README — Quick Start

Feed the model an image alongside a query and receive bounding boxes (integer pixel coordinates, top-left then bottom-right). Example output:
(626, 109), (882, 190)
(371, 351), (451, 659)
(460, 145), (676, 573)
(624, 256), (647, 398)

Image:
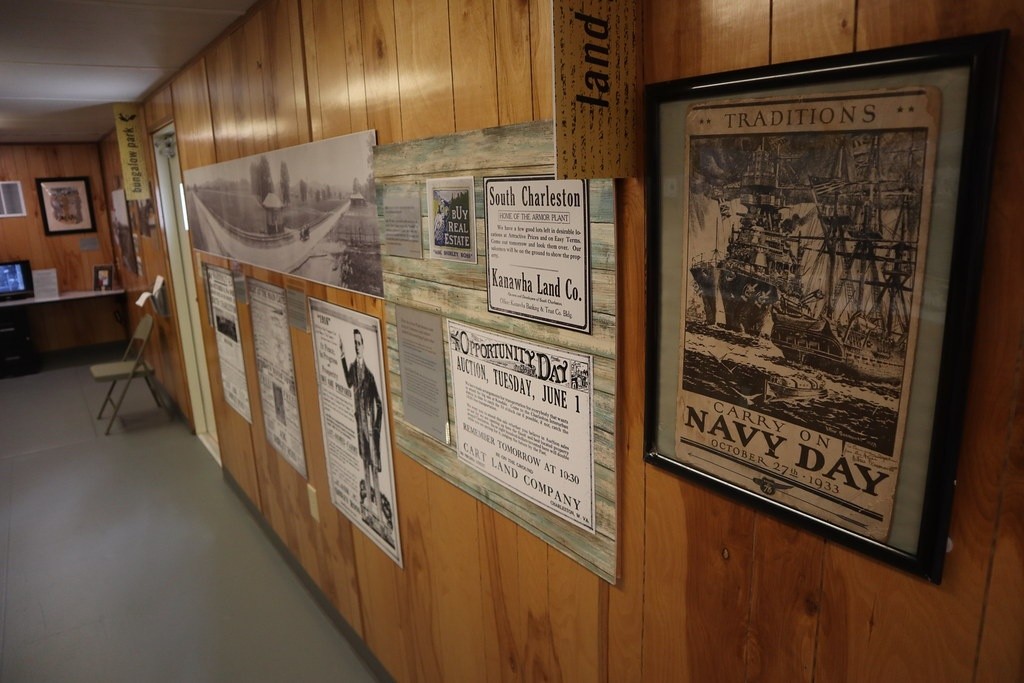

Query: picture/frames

(641, 27), (1012, 586)
(93, 265), (114, 292)
(34, 176), (98, 236)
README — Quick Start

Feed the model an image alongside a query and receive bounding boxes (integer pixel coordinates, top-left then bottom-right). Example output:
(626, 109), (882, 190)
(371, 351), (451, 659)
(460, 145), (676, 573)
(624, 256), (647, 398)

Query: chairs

(88, 312), (175, 437)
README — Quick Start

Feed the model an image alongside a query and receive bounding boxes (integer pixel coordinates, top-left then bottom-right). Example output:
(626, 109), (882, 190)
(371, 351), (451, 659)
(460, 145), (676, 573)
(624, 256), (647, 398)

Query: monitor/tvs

(0, 259), (34, 302)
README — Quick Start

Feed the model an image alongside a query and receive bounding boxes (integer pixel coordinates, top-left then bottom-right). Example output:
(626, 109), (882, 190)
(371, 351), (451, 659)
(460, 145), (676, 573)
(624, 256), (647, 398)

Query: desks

(0, 283), (125, 308)
